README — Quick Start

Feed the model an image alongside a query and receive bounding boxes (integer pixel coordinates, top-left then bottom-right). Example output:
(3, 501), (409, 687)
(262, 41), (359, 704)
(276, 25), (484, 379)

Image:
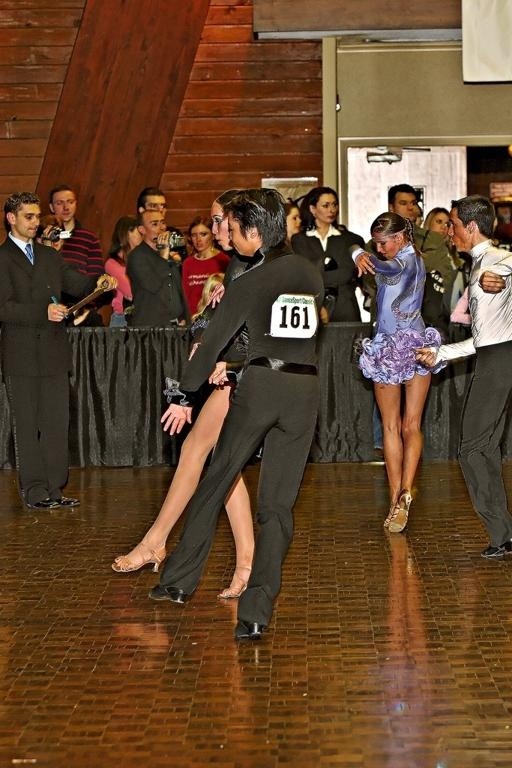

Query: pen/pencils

(51, 296), (69, 320)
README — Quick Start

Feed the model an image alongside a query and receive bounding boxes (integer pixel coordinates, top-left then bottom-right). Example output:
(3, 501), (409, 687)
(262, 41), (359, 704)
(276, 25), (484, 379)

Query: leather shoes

(480, 537), (512, 558)
(148, 583), (190, 604)
(55, 496), (81, 507)
(235, 619), (264, 640)
(27, 497), (61, 509)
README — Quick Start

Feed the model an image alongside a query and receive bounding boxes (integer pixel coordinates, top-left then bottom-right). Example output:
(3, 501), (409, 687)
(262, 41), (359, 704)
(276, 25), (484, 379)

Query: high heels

(219, 567), (252, 599)
(111, 541), (167, 573)
(388, 489), (412, 533)
(383, 503), (409, 529)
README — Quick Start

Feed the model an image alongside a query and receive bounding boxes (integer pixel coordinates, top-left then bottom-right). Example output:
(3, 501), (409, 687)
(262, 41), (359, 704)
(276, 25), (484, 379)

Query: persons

(282, 184), (474, 325)
(413, 194), (512, 558)
(111, 189), (255, 599)
(1, 192), (81, 510)
(348, 213), (447, 534)
(146, 188), (325, 642)
(38, 187), (230, 327)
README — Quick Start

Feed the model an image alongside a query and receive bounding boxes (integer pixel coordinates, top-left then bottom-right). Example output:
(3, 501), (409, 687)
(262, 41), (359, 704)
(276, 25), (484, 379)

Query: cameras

(49, 226), (71, 242)
(168, 231), (186, 249)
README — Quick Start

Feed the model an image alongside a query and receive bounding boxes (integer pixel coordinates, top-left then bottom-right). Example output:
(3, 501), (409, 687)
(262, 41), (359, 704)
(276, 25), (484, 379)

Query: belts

(249, 357), (318, 375)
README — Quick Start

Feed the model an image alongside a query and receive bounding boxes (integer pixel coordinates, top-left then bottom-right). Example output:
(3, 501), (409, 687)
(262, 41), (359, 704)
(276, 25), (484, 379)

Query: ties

(25, 244), (33, 265)
